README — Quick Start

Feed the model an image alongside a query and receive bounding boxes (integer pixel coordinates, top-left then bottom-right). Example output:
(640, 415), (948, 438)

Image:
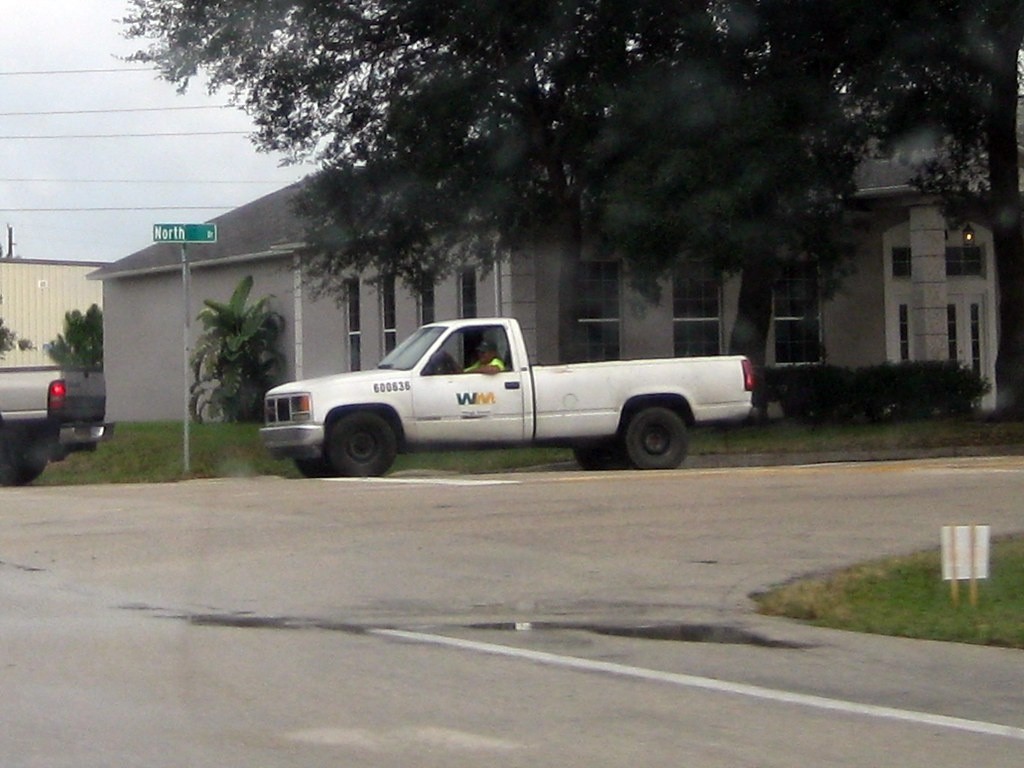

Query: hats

(476, 339), (496, 352)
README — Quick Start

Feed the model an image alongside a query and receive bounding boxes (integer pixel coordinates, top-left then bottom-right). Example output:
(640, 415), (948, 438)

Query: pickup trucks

(0, 366), (105, 485)
(259, 317), (755, 477)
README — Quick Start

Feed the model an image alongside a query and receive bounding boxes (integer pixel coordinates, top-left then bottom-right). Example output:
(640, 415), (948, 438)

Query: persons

(449, 340), (505, 374)
